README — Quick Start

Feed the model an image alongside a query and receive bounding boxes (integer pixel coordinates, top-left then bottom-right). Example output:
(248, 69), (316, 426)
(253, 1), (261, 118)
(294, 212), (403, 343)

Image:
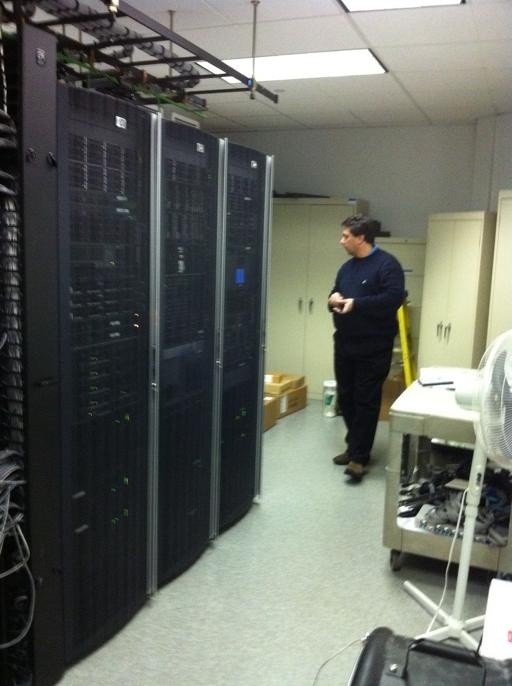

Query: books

(417, 373), (455, 386)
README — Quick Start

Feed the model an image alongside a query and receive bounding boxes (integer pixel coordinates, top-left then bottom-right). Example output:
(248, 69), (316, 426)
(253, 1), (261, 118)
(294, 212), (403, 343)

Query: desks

(380, 367), (511, 580)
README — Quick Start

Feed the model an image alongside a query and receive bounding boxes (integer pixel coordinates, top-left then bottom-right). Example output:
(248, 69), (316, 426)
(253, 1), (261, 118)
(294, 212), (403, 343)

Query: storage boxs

(263, 372), (307, 435)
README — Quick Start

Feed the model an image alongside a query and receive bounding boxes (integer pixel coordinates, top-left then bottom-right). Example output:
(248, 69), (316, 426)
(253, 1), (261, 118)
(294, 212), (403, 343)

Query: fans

(401, 328), (512, 650)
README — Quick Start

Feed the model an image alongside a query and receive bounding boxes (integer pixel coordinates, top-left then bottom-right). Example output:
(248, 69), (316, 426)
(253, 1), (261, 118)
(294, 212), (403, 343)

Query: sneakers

(345, 460), (369, 478)
(333, 452), (370, 465)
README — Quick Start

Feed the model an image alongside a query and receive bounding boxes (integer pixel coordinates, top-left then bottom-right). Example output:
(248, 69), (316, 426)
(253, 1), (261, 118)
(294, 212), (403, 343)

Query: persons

(326, 213), (408, 482)
